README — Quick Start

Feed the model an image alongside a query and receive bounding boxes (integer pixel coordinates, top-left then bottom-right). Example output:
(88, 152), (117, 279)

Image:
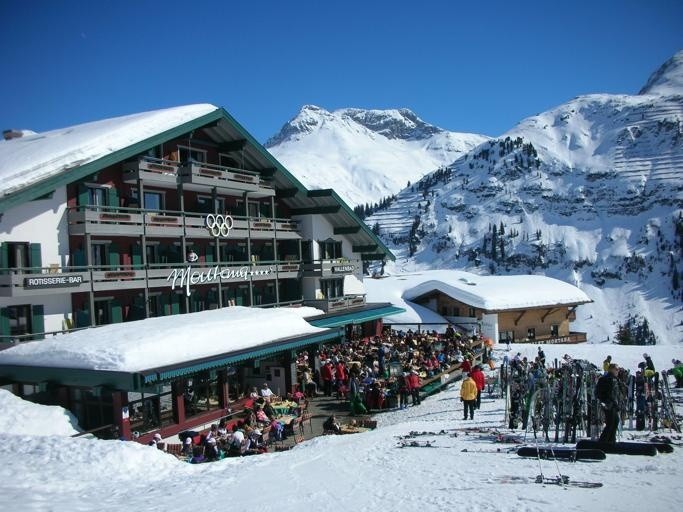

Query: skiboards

(394, 427), (532, 454)
(487, 472), (603, 489)
(499, 358), (682, 443)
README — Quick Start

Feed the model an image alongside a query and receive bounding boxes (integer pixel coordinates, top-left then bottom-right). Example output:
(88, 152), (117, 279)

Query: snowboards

(576, 440), (659, 456)
(517, 447), (606, 460)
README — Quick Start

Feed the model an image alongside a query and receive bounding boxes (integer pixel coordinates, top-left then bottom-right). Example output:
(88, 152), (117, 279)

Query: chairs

(322, 420), (377, 434)
(156, 391), (313, 460)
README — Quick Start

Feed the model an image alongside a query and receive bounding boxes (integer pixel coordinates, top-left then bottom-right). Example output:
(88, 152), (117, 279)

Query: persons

(510, 347), (683, 442)
(133, 383), (304, 463)
(297, 323), (496, 416)
(187, 248), (198, 267)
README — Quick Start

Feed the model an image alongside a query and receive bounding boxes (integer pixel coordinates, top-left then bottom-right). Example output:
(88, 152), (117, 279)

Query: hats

(186, 437), (192, 445)
(208, 438), (216, 443)
(154, 434), (162, 441)
(254, 430), (262, 436)
